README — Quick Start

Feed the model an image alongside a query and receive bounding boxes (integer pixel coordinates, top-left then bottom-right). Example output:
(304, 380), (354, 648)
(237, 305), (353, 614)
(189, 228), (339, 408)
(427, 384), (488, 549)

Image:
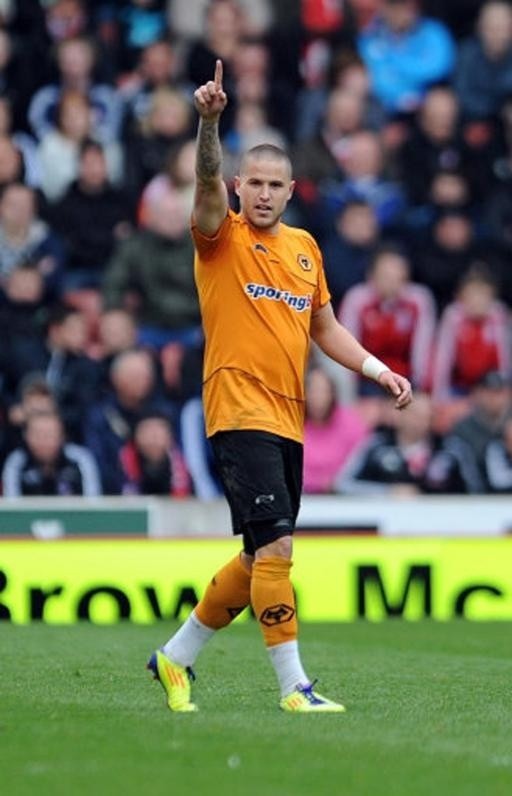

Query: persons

(148, 59), (414, 713)
(1, 1), (511, 497)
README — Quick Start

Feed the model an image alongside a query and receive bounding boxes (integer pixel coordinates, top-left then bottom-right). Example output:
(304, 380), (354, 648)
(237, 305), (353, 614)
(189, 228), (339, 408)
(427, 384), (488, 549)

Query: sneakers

(146, 650), (197, 713)
(279, 687), (345, 714)
